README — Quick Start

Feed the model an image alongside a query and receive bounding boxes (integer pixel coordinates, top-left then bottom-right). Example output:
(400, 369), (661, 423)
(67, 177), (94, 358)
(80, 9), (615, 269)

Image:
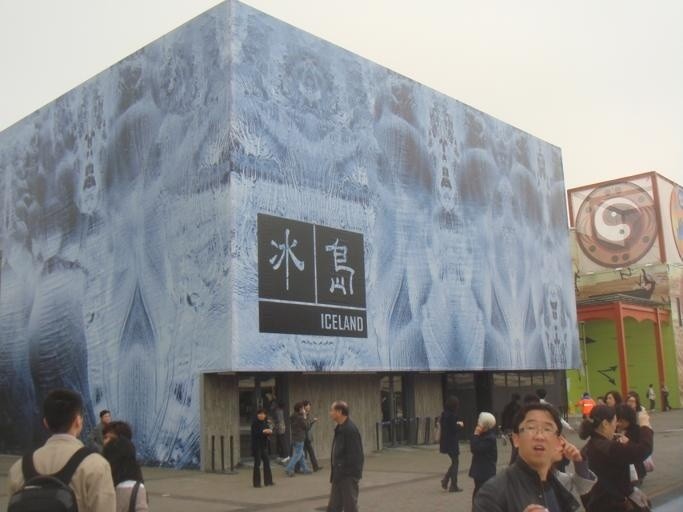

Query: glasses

(519, 425), (560, 437)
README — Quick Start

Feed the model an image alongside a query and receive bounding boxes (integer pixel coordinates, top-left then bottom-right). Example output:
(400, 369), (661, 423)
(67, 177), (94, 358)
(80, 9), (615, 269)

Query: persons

(249, 408), (275, 487)
(325, 399), (364, 512)
(283, 402), (316, 477)
(9, 387), (116, 512)
(268, 387), (291, 464)
(87, 410), (112, 452)
(439, 381), (674, 511)
(99, 420), (132, 458)
(293, 400), (324, 473)
(101, 435), (146, 512)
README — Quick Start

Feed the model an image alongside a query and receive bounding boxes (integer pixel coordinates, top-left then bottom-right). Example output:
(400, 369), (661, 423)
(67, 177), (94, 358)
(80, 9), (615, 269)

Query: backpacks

(6, 446), (96, 511)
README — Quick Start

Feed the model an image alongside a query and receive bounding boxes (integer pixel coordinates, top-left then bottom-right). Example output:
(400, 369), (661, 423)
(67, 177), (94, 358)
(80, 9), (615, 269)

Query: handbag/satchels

(623, 490), (650, 512)
